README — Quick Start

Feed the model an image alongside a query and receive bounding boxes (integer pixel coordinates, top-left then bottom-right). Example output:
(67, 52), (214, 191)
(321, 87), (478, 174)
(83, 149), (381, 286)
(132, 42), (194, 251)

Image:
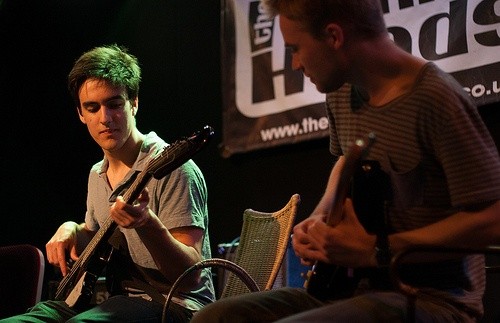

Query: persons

(0, 43), (217, 323)
(187, 0), (500, 323)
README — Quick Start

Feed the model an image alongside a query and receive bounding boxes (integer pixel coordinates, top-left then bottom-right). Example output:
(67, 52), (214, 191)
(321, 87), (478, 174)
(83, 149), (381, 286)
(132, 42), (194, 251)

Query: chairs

(162, 193), (301, 323)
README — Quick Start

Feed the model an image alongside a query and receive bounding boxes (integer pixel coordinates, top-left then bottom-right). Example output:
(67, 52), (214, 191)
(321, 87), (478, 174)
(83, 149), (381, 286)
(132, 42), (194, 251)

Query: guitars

(54, 126), (215, 308)
(303, 133), (385, 300)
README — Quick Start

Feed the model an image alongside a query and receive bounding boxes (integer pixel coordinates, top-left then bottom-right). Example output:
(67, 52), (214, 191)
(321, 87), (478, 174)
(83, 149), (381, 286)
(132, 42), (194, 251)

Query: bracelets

(376, 234), (393, 266)
